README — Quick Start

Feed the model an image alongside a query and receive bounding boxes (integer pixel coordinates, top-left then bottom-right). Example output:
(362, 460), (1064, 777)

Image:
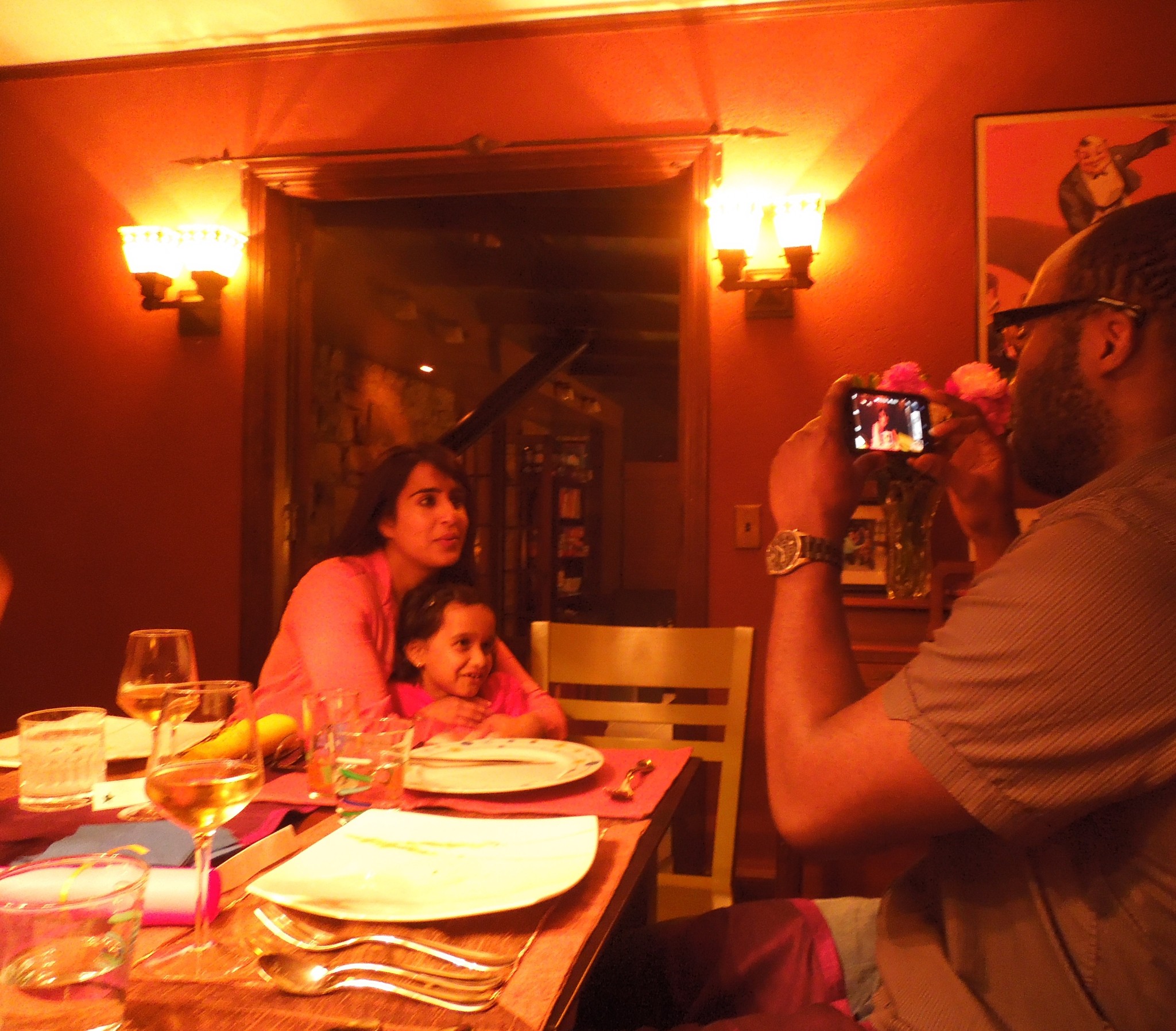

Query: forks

(253, 898), (518, 969)
(607, 758), (654, 799)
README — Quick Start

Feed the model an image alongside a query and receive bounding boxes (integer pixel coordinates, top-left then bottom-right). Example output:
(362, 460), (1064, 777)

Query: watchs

(765, 529), (842, 576)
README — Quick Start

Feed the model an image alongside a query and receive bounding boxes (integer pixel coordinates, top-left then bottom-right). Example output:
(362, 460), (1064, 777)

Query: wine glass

(135, 679), (266, 980)
(114, 630), (202, 793)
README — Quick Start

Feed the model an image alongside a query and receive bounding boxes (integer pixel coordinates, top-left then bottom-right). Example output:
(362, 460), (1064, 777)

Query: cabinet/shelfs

(522, 434), (603, 626)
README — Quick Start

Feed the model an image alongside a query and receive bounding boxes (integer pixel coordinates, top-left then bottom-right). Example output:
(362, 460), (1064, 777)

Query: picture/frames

(974, 100), (1176, 364)
(838, 498), (890, 596)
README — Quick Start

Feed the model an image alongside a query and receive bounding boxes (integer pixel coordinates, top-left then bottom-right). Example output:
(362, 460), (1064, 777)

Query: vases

(886, 474), (943, 593)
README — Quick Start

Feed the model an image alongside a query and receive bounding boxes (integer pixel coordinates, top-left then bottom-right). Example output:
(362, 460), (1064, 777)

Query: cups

(1, 854), (146, 1031)
(14, 706), (106, 811)
(335, 716), (417, 806)
(301, 690), (362, 803)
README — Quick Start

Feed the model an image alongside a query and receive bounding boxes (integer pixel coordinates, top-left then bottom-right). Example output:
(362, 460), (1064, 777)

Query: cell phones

(844, 388), (933, 461)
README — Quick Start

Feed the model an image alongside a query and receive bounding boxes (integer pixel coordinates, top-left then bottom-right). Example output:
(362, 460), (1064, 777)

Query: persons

(577, 190), (1176, 1031)
(221, 442), (568, 745)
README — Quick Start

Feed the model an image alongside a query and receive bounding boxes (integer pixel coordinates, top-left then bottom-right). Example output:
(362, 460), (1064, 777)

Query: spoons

(255, 951), (505, 1015)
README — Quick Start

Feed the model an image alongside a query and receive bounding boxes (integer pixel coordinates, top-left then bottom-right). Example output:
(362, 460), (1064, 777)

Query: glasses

(987, 296), (1141, 381)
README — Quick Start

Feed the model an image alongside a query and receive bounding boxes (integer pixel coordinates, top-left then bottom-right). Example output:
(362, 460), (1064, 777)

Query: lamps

(703, 180), (824, 323)
(117, 223), (252, 335)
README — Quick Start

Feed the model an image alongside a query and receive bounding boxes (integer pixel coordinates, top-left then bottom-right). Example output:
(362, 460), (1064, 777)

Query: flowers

(862, 352), (1011, 585)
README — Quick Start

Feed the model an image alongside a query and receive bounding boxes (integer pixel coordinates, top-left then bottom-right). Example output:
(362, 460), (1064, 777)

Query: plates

(0, 711), (227, 764)
(245, 807), (601, 926)
(400, 737), (605, 793)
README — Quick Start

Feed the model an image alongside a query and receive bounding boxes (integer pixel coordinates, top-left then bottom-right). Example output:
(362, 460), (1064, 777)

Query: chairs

(528, 621), (756, 925)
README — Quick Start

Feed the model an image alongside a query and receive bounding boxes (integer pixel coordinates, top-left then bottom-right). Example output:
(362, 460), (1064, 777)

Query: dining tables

(0, 711), (701, 1031)
